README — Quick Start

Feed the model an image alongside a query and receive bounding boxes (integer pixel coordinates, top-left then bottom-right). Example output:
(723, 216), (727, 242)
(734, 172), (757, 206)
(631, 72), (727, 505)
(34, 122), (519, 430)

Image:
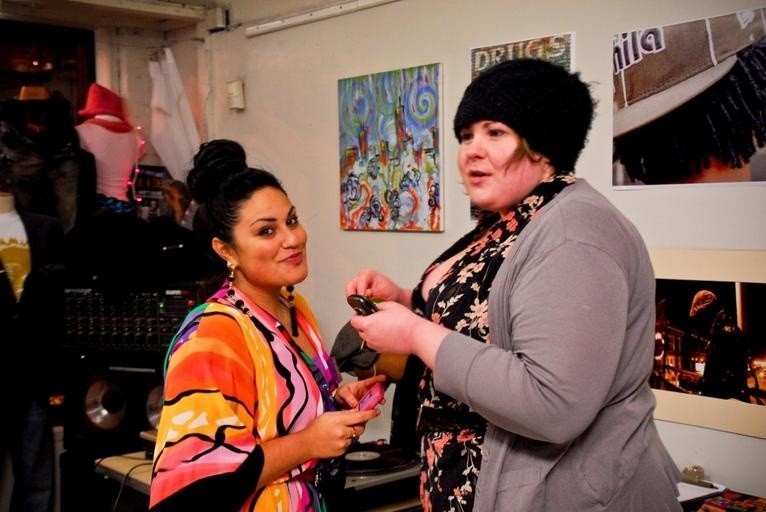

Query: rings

(351, 426), (358, 440)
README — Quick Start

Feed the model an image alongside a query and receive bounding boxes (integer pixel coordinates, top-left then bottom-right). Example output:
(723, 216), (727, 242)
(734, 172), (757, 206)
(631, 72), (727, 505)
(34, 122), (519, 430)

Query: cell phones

(347, 293), (377, 314)
(354, 381), (384, 414)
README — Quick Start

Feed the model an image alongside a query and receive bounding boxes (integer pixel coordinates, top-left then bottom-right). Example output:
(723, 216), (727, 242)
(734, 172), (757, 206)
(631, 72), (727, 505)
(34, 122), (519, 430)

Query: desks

(92, 429), (424, 512)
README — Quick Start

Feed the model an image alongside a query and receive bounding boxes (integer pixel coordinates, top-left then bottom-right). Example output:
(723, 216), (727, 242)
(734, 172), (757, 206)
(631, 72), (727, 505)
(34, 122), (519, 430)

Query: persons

(680, 291), (751, 401)
(148, 137), (389, 512)
(160, 140), (247, 300)
(345, 58), (684, 512)
(615, 6), (765, 185)
(0, 82), (144, 510)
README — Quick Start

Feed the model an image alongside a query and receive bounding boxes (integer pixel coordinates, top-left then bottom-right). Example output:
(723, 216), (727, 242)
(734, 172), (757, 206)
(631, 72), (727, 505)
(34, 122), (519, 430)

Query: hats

(689, 289), (717, 317)
(77, 83), (125, 121)
(453, 58), (593, 171)
